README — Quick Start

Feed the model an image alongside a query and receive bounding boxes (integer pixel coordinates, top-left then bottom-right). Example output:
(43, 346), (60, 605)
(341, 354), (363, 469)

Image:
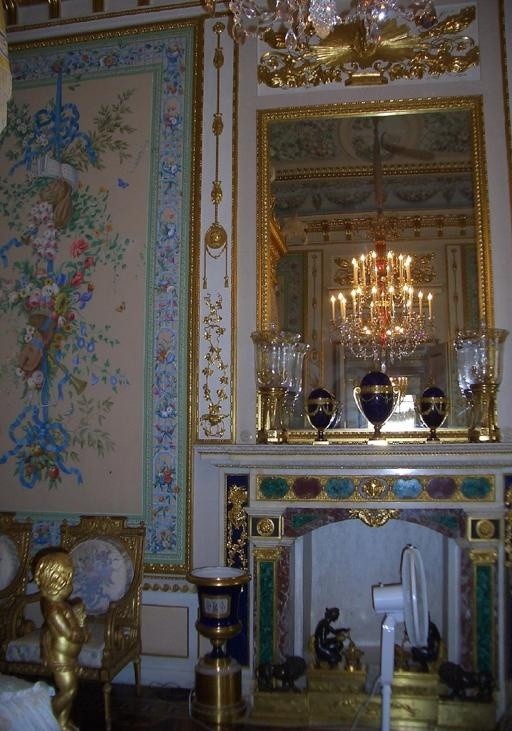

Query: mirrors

(255, 95), (500, 443)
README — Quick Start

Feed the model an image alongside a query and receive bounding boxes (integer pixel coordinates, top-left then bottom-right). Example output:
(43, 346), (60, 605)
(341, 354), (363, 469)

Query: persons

(315, 607), (346, 668)
(31, 551), (89, 731)
(405, 612), (441, 673)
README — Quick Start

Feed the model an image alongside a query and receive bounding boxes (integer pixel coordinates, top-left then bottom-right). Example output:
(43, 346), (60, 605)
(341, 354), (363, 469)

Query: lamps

(328, 117), (439, 374)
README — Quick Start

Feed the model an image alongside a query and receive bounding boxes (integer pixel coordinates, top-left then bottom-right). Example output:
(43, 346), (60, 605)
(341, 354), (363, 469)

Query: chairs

(0, 515), (146, 731)
(0, 512), (34, 646)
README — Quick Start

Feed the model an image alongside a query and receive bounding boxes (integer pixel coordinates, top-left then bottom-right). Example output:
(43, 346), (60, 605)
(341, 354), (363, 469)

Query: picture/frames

(0, 15), (205, 577)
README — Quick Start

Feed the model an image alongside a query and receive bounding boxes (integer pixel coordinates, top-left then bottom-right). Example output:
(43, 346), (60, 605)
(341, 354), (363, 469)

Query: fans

(372, 543), (430, 731)
